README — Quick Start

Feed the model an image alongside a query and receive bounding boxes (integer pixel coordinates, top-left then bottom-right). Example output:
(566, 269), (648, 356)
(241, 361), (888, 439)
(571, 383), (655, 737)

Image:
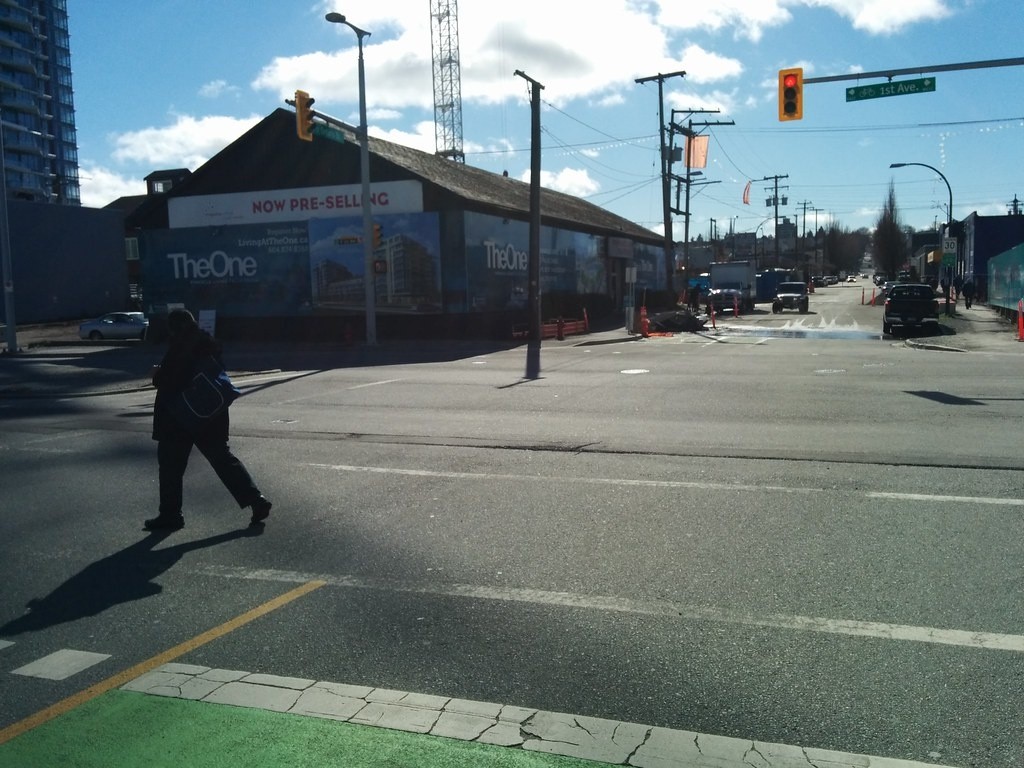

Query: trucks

(707, 261), (756, 314)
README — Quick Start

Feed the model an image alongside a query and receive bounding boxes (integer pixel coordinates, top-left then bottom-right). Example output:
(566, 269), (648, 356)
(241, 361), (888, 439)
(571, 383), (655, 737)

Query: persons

(143, 309), (272, 530)
(941, 275), (951, 294)
(929, 276), (938, 291)
(689, 283), (701, 311)
(953, 275), (963, 299)
(963, 278), (975, 309)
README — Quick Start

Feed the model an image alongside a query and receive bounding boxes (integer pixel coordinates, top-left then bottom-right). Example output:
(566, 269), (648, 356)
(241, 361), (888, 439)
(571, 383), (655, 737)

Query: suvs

(772, 282), (809, 314)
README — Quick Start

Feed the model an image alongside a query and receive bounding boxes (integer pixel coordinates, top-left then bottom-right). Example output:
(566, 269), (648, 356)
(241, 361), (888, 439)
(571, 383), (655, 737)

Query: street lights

(325, 13), (377, 343)
(890, 163), (954, 315)
(754, 216), (786, 271)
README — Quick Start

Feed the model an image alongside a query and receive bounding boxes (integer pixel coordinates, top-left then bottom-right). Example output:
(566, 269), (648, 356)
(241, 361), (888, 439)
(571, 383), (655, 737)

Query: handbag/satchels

(165, 346), (242, 432)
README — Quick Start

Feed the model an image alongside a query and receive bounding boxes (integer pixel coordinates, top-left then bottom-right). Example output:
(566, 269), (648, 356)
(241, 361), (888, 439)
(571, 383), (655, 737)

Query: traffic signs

(845, 76), (936, 102)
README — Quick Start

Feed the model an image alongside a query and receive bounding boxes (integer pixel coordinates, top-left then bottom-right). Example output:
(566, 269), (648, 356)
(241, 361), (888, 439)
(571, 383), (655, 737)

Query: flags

(743, 180), (752, 205)
(684, 135), (709, 168)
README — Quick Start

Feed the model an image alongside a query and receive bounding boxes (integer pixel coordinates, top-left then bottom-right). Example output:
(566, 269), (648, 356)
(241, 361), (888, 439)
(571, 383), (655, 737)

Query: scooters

(878, 281), (888, 305)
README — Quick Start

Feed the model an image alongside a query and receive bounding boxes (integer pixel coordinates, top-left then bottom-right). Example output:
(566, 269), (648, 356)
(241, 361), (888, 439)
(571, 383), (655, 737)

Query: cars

(811, 276), (838, 287)
(862, 274), (869, 279)
(847, 275), (856, 282)
(79, 312), (149, 340)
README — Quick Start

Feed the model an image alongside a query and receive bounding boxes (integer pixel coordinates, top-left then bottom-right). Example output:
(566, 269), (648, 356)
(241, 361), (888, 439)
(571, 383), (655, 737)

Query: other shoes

(145, 510), (184, 528)
(251, 495), (272, 522)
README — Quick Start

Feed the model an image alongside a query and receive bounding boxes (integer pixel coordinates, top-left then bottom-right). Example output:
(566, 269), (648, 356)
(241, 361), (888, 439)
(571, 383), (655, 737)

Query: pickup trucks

(872, 270), (940, 333)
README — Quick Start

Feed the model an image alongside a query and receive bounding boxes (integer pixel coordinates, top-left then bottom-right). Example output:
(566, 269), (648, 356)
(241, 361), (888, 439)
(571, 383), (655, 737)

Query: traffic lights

(295, 90), (319, 142)
(556, 316), (566, 340)
(779, 68), (803, 120)
